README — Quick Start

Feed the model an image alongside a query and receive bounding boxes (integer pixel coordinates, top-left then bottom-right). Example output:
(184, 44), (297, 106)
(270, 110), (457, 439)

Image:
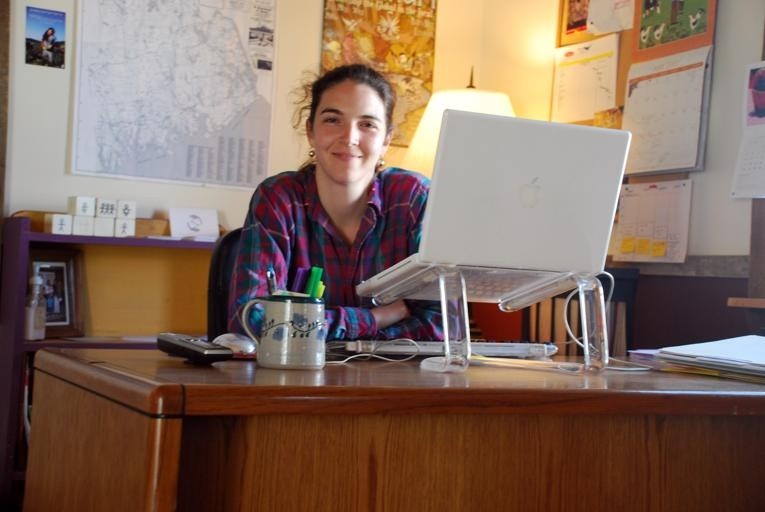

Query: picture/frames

(25, 241), (89, 339)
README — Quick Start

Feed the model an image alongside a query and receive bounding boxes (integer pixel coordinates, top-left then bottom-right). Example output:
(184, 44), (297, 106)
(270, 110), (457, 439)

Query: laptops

(355, 109), (631, 304)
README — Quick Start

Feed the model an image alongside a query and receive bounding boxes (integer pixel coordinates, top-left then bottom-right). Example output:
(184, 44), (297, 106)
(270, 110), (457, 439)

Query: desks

(19, 346), (763, 512)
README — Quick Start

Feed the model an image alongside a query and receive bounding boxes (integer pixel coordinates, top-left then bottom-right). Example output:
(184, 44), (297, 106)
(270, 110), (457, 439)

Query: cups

(241, 295), (326, 372)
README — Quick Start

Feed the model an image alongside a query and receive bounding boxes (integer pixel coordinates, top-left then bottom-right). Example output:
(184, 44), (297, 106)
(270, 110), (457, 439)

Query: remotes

(158, 332), (232, 366)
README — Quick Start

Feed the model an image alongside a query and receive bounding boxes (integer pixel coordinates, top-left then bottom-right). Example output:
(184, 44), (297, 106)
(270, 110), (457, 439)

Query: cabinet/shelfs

(2, 206), (227, 512)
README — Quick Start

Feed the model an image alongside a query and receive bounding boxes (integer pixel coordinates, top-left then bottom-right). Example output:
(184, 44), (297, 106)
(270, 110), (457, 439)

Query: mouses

(214, 332), (255, 354)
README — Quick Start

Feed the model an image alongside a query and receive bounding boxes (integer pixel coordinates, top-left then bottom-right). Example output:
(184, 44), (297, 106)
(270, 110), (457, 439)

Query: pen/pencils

(265, 263), (326, 300)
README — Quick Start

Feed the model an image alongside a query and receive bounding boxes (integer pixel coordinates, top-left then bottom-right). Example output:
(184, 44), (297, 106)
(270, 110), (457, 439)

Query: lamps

(407, 65), (522, 166)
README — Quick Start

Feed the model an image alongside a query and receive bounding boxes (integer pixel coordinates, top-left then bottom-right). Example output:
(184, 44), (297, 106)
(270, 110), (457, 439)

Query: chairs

(206, 224), (245, 345)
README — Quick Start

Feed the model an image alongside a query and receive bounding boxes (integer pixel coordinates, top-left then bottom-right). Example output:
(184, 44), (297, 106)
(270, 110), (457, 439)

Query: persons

(42, 279), (63, 316)
(41, 28), (57, 63)
(229, 65), (459, 341)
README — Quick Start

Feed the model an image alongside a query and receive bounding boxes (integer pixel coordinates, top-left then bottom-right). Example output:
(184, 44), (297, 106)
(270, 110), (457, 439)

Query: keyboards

(329, 339), (557, 360)
